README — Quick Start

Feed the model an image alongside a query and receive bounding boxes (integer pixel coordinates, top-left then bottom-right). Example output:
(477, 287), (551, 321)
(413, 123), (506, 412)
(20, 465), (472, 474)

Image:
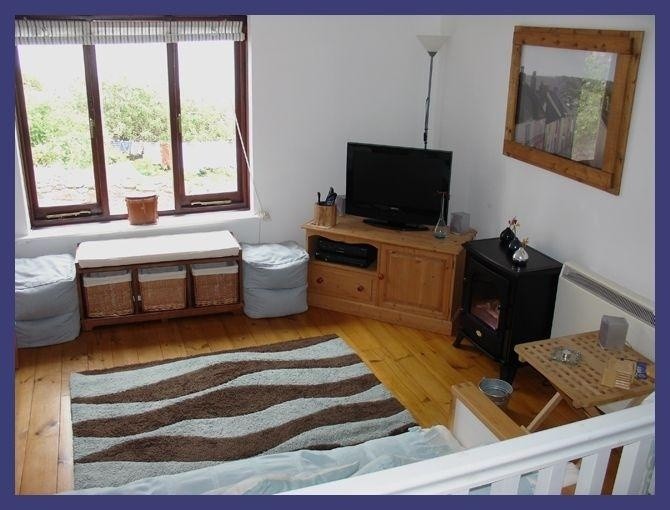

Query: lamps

(418, 34), (450, 151)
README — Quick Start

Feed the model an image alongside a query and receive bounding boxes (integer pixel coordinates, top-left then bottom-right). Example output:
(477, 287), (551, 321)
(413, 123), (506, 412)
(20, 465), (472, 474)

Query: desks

(514, 330), (655, 433)
(73, 231), (243, 332)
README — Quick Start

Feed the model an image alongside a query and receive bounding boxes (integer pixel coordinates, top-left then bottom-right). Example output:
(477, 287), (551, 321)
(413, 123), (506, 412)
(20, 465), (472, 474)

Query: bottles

(499, 227), (529, 264)
(434, 192), (449, 239)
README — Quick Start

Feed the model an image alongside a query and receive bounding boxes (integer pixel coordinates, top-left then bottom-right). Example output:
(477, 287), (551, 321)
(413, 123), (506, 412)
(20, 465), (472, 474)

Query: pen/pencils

(315, 187), (338, 204)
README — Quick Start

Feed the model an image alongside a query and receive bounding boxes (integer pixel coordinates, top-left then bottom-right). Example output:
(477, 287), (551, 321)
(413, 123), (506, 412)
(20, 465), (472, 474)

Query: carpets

(68, 333), (421, 489)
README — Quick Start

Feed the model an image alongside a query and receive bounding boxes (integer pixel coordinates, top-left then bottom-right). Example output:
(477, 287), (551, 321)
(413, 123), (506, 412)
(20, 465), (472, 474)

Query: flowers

(508, 215), (531, 248)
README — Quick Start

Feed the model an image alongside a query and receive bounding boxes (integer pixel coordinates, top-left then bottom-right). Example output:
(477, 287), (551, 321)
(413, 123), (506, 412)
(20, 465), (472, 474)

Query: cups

(313, 201), (336, 227)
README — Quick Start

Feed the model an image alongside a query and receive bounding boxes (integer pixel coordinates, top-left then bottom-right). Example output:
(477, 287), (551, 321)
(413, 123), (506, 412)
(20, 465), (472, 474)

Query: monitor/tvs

(345, 142), (453, 231)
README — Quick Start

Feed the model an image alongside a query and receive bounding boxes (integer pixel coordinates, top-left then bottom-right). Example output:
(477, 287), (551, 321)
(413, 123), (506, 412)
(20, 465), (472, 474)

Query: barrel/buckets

(478, 377), (514, 412)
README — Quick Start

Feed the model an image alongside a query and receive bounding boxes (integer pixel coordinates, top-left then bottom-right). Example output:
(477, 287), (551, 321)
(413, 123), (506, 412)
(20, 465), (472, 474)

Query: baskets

(84, 281), (135, 319)
(139, 279), (186, 312)
(193, 273), (239, 308)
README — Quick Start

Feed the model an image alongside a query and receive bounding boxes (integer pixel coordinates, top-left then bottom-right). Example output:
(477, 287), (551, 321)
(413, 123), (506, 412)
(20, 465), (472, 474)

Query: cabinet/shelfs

(452, 237), (563, 387)
(300, 211), (476, 337)
(501, 25), (645, 197)
